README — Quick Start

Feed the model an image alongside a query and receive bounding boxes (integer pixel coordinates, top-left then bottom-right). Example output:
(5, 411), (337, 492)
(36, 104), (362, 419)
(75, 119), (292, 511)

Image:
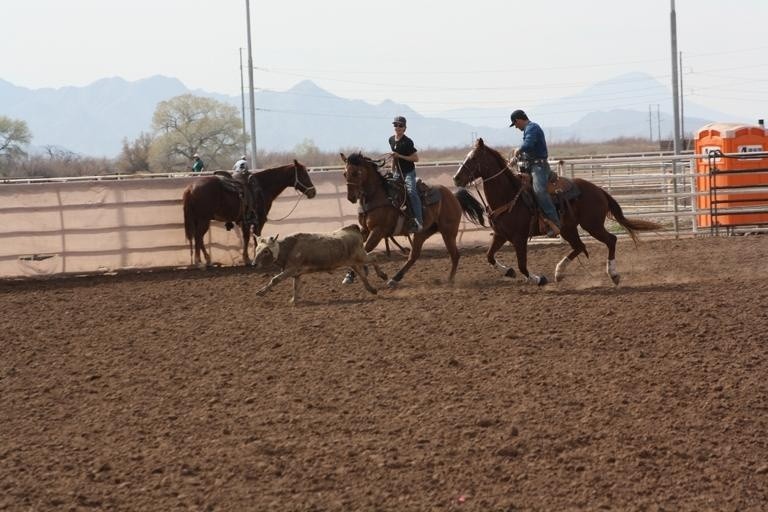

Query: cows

(250, 223), (389, 306)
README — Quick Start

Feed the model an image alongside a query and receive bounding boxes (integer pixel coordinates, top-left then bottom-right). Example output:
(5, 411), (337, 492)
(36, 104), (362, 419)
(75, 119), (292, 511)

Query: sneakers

(409, 218), (424, 230)
(545, 226), (561, 238)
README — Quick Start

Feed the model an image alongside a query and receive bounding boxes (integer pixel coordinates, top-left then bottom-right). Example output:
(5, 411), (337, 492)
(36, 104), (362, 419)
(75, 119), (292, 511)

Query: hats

(392, 116), (406, 123)
(509, 110), (526, 127)
(193, 154), (199, 158)
(241, 156), (247, 159)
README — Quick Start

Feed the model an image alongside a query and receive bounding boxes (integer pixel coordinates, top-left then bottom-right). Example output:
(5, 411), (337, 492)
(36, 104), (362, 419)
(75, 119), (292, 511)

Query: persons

(232, 155), (248, 170)
(191, 154), (205, 176)
(388, 116), (424, 227)
(507, 109), (562, 237)
(235, 163), (246, 172)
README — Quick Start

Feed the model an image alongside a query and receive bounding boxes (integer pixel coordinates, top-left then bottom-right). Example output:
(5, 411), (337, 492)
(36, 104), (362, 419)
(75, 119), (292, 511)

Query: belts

(531, 159), (544, 164)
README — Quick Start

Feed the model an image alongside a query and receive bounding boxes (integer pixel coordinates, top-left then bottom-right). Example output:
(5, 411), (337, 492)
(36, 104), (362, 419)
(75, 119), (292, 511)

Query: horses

(340, 150), (489, 290)
(182, 158), (316, 268)
(452, 137), (664, 286)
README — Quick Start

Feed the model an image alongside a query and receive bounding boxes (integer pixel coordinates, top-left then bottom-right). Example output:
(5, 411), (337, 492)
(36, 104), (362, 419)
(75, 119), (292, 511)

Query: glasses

(394, 124), (404, 127)
